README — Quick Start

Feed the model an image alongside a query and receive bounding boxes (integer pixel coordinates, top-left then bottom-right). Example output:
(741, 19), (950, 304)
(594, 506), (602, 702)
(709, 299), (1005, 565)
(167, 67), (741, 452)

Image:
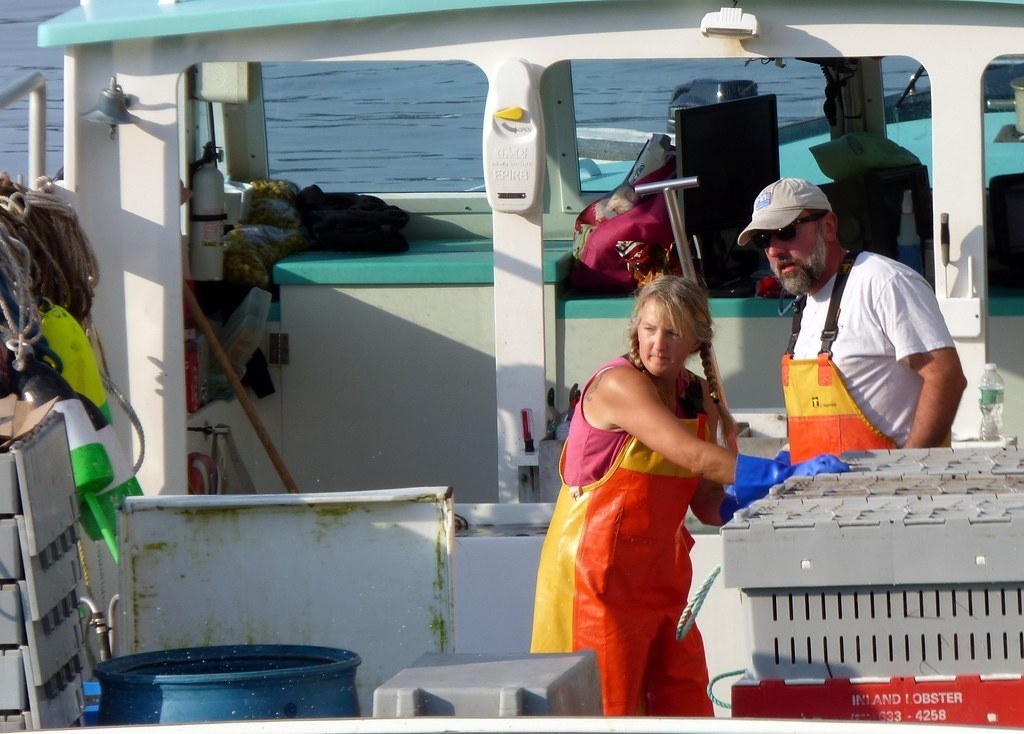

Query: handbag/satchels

(570, 146), (681, 298)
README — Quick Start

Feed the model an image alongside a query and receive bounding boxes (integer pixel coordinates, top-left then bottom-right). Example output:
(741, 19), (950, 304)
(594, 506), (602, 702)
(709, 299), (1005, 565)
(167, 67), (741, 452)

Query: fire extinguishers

(191, 143), (226, 281)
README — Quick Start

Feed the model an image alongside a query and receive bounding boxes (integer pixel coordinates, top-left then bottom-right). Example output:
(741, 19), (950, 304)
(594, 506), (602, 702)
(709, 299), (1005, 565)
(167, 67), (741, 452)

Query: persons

(530, 276), (850, 717)
(737, 177), (967, 466)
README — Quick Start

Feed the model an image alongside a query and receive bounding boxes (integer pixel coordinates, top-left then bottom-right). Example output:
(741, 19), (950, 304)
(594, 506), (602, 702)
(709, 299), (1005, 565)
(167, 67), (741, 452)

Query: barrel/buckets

(92, 644), (363, 727)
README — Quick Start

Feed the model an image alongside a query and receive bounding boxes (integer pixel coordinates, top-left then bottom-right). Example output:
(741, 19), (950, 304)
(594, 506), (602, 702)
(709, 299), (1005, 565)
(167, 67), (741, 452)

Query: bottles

(979, 362), (1006, 441)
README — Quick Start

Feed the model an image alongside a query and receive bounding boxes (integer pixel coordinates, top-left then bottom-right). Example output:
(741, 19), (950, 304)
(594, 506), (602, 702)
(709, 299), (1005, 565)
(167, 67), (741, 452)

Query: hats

(737, 177), (832, 246)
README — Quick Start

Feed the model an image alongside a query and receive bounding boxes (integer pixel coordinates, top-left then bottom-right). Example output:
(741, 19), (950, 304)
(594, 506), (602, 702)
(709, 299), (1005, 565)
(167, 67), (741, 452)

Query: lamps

(700, 7), (761, 38)
(78, 76), (141, 143)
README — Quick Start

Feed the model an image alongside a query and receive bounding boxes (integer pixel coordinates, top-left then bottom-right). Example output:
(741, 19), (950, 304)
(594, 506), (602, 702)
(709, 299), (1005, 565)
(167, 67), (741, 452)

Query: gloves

(720, 450), (797, 524)
(735, 454), (849, 508)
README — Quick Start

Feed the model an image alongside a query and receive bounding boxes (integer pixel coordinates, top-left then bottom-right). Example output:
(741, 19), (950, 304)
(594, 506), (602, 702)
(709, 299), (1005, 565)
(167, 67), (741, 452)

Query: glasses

(750, 212), (824, 250)
